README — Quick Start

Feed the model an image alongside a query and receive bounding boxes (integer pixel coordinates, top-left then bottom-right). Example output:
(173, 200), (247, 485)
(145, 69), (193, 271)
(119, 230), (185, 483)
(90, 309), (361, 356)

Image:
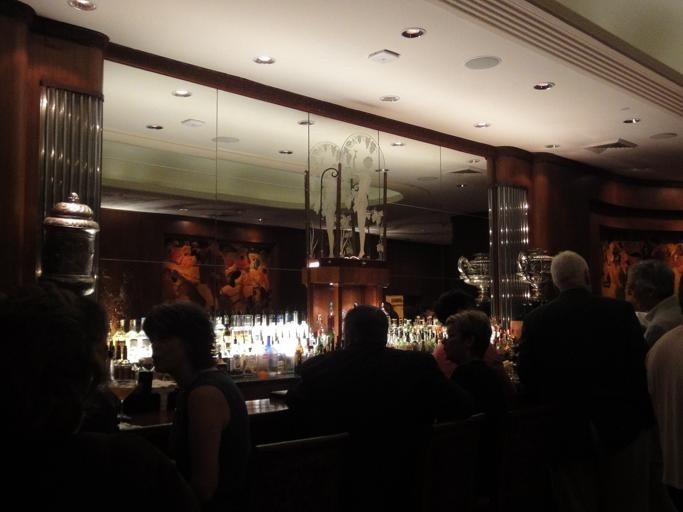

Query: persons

(288, 304), (470, 510)
(626, 260), (683, 347)
(517, 248), (664, 512)
(439, 309), (533, 512)
(644, 269), (683, 510)
(142, 299), (250, 512)
(0, 283), (122, 511)
(431, 289), (520, 393)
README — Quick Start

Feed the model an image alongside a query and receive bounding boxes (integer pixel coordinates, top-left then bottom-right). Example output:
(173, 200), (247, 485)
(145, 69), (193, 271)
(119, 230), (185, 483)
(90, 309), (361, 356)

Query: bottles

(376, 301), (500, 354)
(101, 299), (341, 386)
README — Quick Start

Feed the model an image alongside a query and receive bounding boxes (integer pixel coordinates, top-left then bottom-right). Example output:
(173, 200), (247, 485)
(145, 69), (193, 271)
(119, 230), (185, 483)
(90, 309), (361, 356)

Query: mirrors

(97, 53), (494, 377)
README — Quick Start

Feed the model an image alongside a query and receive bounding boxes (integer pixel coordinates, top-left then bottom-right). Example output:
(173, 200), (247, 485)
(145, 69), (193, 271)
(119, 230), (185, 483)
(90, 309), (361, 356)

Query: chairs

(249, 429), (358, 512)
(425, 408), (492, 511)
